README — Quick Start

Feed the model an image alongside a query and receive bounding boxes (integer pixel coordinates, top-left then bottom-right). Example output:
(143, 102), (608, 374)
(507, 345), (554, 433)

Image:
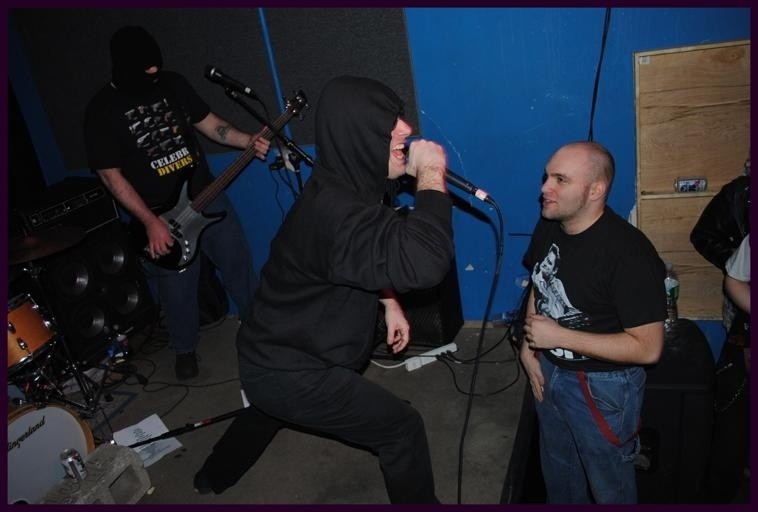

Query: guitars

(131, 88), (312, 273)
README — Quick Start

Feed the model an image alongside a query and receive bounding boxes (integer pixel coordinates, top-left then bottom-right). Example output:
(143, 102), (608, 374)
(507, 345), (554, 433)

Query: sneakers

(175, 351), (201, 377)
(194, 467), (212, 495)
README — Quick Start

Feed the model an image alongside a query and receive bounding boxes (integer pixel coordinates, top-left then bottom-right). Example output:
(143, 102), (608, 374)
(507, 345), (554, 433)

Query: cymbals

(6, 229), (81, 266)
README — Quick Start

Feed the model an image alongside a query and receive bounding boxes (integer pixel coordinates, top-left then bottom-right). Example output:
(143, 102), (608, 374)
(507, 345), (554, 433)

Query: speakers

(395, 264), (464, 348)
(24, 230), (159, 372)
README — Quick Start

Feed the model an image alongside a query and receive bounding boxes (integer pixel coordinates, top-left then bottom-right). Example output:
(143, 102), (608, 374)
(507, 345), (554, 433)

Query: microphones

(203, 64), (262, 103)
(405, 149), (494, 206)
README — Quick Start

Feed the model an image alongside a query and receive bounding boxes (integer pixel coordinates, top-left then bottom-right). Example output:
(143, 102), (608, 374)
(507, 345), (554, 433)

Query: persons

(82, 25), (271, 380)
(518, 140), (670, 504)
(722, 234), (752, 315)
(688, 158), (752, 480)
(192, 74), (457, 504)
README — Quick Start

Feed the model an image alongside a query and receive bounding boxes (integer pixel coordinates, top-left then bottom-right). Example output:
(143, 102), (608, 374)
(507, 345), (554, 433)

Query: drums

(7, 292), (57, 375)
(6, 399), (95, 505)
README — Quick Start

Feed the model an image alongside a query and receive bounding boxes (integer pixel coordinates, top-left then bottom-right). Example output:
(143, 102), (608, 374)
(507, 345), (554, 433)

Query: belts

(540, 349), (637, 372)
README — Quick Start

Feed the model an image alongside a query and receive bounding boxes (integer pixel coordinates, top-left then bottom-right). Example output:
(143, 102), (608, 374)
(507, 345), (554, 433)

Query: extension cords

(403, 342), (459, 373)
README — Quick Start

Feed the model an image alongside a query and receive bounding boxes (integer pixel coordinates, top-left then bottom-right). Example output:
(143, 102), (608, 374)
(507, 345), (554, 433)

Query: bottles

(665, 262), (678, 324)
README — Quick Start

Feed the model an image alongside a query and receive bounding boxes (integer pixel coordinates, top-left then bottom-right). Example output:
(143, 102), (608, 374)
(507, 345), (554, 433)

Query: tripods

(15, 260), (149, 447)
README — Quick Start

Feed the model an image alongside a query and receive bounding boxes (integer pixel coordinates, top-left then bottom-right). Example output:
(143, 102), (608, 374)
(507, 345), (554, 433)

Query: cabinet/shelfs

(634, 37), (752, 327)
(633, 317), (717, 503)
